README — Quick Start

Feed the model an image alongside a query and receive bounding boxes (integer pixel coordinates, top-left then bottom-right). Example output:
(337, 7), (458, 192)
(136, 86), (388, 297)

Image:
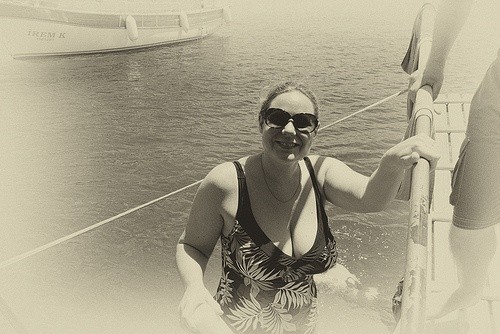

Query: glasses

(261, 107), (321, 133)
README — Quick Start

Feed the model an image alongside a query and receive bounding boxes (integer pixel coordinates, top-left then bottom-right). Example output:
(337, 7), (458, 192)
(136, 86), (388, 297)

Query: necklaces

(258, 156), (302, 202)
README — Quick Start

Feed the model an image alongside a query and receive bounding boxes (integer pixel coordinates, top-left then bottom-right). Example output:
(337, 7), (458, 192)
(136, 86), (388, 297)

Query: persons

(174, 81), (443, 334)
(407, 2), (499, 306)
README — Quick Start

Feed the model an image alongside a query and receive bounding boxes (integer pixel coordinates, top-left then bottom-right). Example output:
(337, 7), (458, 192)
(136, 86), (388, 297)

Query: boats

(0, 1), (225, 59)
